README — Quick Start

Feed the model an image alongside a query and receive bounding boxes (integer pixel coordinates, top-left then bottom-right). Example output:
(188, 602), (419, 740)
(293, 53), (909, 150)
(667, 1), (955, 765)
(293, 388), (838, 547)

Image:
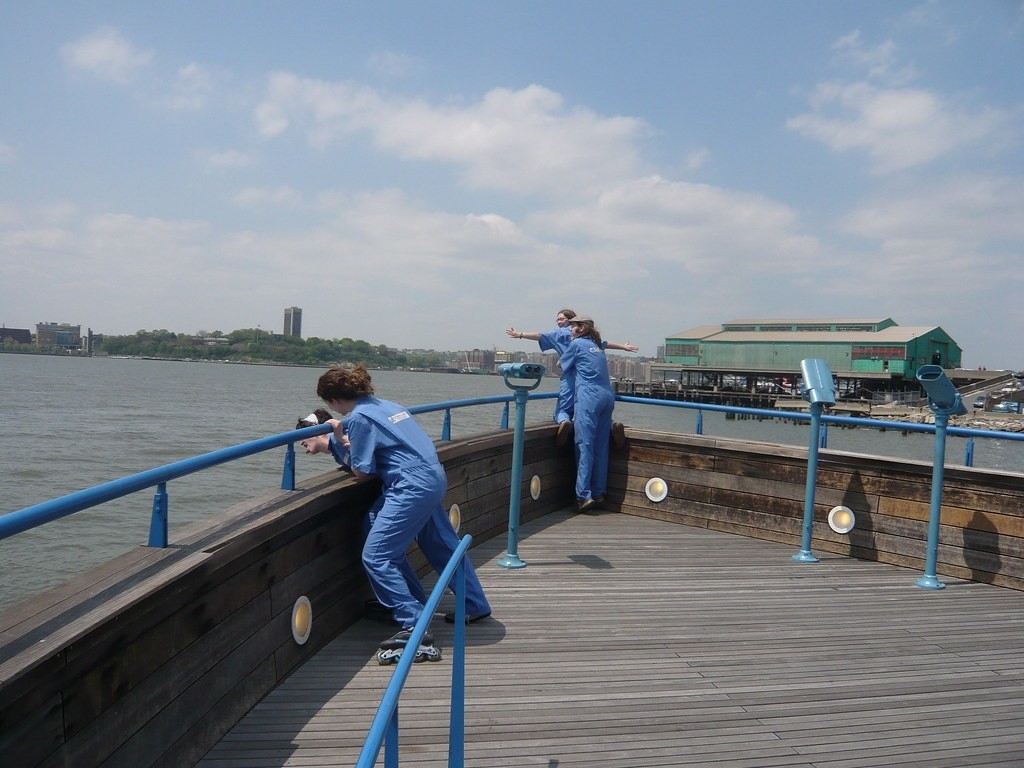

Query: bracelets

(519, 332), (523, 340)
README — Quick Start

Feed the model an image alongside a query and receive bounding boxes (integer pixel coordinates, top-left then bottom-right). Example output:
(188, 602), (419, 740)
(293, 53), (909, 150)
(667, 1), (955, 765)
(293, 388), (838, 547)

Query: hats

(566, 315), (594, 327)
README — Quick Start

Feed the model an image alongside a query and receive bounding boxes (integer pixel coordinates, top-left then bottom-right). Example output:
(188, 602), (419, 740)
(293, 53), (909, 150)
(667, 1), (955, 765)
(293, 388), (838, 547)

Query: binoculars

(918, 364), (968, 412)
(803, 360), (840, 406)
(497, 361), (546, 379)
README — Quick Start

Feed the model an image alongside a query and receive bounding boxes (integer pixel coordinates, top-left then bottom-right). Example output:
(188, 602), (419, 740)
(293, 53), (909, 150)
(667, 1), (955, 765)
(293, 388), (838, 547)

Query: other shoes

(612, 423), (625, 449)
(576, 497), (596, 515)
(446, 609), (490, 624)
(557, 421), (571, 444)
(595, 496), (605, 506)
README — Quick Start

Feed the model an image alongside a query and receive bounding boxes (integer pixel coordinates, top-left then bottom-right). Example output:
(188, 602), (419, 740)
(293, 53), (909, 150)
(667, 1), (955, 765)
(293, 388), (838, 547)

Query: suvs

(1002, 382), (1022, 397)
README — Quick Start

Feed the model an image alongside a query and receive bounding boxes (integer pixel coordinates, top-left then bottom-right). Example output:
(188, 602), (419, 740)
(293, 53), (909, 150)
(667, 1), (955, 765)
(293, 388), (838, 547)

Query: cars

(989, 390), (1003, 403)
(973, 395), (986, 408)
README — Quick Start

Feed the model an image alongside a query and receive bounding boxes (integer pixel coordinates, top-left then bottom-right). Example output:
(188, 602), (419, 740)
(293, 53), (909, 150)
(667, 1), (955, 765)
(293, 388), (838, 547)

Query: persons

(296, 366), (492, 651)
(505, 308), (639, 514)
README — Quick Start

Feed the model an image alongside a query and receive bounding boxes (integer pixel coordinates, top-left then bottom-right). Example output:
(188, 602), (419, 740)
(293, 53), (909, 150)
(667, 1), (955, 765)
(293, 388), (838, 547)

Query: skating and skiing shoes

(377, 624), (442, 666)
(363, 598), (395, 623)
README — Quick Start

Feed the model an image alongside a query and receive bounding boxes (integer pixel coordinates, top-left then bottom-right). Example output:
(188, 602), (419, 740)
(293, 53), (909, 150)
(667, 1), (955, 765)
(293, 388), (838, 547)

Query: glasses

(555, 317), (569, 322)
